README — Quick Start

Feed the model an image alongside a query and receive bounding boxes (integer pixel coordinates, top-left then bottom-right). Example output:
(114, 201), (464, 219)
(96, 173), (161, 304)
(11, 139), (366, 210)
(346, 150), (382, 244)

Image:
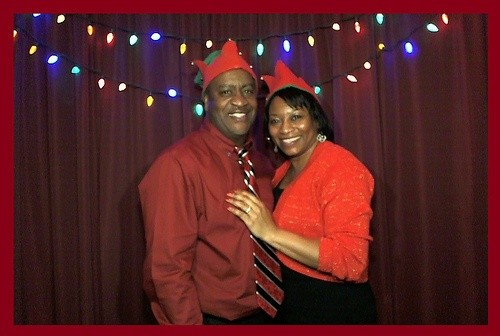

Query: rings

(244, 207), (251, 214)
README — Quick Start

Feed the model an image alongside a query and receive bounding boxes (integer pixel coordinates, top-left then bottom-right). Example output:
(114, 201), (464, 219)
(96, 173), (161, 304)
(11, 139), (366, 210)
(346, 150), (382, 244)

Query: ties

(233, 141), (284, 319)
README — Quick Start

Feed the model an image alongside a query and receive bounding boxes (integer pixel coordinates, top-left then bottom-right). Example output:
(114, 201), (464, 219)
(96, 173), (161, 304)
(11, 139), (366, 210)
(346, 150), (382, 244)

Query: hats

(260, 59), (321, 106)
(193, 39), (259, 95)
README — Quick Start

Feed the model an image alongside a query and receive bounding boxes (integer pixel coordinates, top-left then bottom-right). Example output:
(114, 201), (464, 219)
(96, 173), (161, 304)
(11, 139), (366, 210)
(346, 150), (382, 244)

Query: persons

(224, 60), (376, 325)
(137, 43), (284, 325)
